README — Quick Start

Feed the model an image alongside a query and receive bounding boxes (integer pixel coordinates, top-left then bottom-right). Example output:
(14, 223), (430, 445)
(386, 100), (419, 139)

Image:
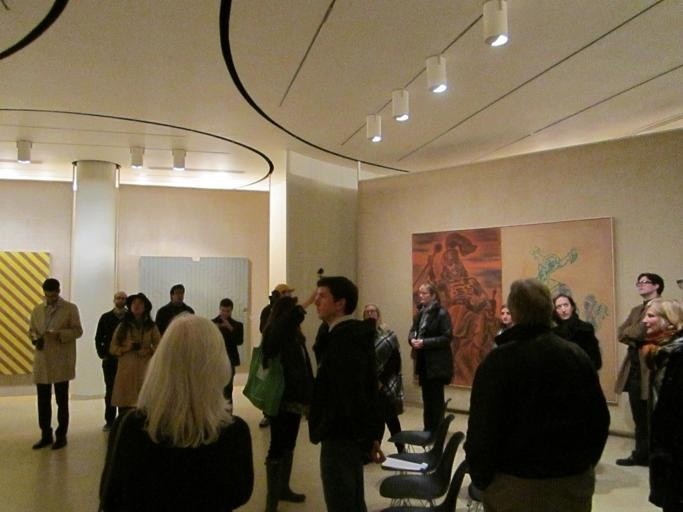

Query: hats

(275, 284), (294, 293)
(127, 293), (152, 311)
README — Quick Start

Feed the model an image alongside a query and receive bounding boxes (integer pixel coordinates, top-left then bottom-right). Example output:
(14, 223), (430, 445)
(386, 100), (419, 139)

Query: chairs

(379, 397), (468, 512)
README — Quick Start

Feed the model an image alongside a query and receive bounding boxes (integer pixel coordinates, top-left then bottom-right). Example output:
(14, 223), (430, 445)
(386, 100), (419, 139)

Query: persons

(550, 293), (601, 374)
(27, 278), (83, 450)
(642, 297), (681, 511)
(492, 304), (513, 351)
(614, 273), (667, 468)
(465, 279), (608, 512)
(98, 314), (254, 512)
(260, 273), (406, 512)
(95, 284), (247, 431)
(408, 281), (455, 434)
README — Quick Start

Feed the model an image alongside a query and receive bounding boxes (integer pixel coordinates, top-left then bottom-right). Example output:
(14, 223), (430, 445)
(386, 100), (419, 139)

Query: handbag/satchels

(243, 345), (286, 417)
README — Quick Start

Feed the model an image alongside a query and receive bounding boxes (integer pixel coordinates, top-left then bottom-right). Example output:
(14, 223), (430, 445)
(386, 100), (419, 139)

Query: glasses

(636, 281), (652, 285)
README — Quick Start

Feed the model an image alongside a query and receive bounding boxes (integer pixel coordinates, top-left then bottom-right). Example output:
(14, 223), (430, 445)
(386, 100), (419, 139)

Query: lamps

(16, 139), (32, 164)
(425, 56), (448, 93)
(172, 148), (186, 171)
(483, 0), (508, 47)
(366, 114), (382, 142)
(391, 89), (410, 122)
(130, 145), (146, 168)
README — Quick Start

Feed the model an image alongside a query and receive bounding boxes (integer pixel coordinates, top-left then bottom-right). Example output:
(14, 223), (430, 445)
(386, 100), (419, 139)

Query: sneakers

(265, 492), (305, 512)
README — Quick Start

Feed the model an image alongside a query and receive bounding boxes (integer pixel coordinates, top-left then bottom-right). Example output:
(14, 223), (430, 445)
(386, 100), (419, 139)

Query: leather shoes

(616, 456), (647, 466)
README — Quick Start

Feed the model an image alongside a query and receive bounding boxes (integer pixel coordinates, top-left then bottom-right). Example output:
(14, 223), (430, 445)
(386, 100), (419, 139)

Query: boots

(33, 428), (66, 450)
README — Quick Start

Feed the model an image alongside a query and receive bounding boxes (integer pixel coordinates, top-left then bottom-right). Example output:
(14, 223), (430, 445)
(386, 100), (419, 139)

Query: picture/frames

(411, 216), (619, 404)
